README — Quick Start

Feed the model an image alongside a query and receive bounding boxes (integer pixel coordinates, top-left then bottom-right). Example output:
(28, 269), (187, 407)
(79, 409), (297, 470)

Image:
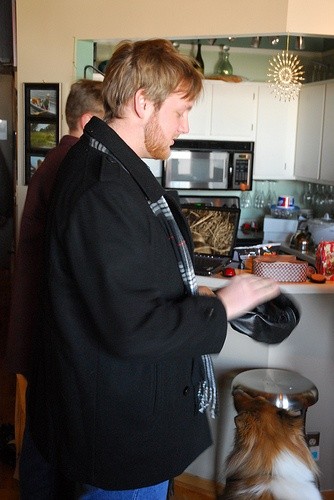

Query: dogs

(222, 389), (325, 500)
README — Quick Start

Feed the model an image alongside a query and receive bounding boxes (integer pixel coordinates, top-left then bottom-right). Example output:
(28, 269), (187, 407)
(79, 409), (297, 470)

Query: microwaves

(161, 138), (255, 191)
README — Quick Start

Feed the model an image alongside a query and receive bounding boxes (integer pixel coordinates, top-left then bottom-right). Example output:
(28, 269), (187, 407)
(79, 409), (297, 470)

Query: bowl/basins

(278, 196), (294, 209)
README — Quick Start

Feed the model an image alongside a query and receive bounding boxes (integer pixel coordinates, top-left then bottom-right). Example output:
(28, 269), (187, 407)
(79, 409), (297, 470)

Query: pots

(214, 285), (300, 347)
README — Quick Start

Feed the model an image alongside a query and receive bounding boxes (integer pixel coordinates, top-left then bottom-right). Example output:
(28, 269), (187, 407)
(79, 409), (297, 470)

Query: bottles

(304, 182), (334, 219)
(216, 52), (233, 77)
(193, 43), (204, 76)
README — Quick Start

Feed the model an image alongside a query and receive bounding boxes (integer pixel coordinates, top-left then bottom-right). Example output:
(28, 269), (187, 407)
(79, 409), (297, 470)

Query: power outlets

(306, 432), (320, 447)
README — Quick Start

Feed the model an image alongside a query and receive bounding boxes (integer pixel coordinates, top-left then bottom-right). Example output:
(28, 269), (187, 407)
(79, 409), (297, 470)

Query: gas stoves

(177, 195), (241, 288)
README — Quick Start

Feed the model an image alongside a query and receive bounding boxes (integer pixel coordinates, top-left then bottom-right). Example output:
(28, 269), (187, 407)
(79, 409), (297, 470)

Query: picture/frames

(23, 80), (61, 187)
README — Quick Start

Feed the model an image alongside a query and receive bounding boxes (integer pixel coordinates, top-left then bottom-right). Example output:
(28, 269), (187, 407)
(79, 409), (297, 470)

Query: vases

(193, 43), (204, 75)
(217, 52), (233, 76)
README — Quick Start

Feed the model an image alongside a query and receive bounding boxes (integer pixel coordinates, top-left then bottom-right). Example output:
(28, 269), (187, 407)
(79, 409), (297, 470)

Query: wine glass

(239, 179), (279, 209)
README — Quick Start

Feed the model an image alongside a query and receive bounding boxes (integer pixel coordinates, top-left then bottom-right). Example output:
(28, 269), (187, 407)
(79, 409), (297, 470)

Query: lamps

(250, 36), (262, 49)
(294, 36), (305, 51)
(172, 37), (238, 48)
(269, 35), (280, 45)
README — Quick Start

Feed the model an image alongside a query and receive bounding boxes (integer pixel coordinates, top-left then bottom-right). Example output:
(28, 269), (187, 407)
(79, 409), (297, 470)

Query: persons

(6, 78), (105, 500)
(18, 38), (280, 500)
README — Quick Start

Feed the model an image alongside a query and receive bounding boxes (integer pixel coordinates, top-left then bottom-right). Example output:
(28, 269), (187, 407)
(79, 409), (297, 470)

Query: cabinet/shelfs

(140, 80), (334, 187)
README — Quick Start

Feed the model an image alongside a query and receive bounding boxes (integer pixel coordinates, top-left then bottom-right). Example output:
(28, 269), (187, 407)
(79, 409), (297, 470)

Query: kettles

(288, 221), (315, 253)
